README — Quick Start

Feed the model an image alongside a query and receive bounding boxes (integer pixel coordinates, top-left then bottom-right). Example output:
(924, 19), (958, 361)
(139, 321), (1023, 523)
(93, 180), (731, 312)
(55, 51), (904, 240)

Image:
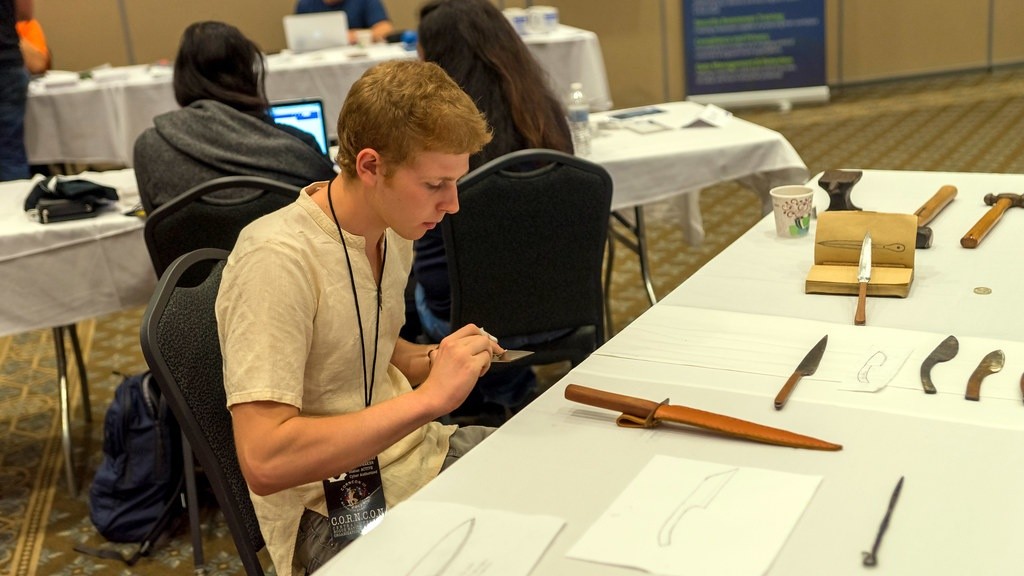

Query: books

(806, 210), (918, 299)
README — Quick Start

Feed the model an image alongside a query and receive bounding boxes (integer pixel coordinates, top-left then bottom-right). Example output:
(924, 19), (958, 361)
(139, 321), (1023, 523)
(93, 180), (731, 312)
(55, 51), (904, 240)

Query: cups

(504, 5), (558, 35)
(769, 185), (814, 240)
(355, 30), (371, 50)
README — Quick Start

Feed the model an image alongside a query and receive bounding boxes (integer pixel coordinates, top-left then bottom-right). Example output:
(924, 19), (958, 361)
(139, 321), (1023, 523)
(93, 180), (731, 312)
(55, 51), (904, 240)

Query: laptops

(283, 11), (349, 52)
(266, 97), (330, 166)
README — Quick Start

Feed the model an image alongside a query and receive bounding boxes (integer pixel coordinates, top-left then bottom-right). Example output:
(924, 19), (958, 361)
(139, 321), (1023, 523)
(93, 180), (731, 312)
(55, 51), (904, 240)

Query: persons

(215, 60), (509, 576)
(294, 0), (394, 44)
(134, 21), (339, 218)
(409, 0), (583, 417)
(0, 0), (52, 184)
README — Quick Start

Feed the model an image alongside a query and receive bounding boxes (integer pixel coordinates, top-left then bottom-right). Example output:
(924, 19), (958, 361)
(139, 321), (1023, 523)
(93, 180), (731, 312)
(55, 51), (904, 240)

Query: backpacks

(71, 369), (199, 565)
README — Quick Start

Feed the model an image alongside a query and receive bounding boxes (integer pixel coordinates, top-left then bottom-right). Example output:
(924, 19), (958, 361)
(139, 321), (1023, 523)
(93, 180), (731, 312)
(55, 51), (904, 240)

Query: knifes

(855, 231), (872, 325)
(920, 335), (959, 394)
(774, 335), (829, 411)
(965, 349), (1005, 401)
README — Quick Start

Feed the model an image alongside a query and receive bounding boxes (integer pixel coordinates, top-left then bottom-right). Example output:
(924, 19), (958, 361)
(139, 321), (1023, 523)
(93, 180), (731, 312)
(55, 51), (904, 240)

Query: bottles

(565, 81), (589, 156)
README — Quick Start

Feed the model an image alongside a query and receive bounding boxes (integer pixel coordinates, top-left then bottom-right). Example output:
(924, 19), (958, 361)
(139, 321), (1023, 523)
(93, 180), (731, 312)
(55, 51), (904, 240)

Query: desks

(0, 99), (805, 497)
(303, 169), (1024, 576)
(22, 18), (613, 176)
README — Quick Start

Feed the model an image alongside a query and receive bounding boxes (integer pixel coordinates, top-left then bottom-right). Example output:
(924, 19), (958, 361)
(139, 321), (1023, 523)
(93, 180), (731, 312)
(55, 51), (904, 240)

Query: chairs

(145, 177), (308, 571)
(414, 146), (614, 430)
(137, 247), (280, 576)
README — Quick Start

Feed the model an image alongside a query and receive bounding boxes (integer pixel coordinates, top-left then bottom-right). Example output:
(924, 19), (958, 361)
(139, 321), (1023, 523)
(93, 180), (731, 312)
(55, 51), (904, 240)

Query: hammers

(960, 193), (1024, 249)
(913, 185), (958, 249)
